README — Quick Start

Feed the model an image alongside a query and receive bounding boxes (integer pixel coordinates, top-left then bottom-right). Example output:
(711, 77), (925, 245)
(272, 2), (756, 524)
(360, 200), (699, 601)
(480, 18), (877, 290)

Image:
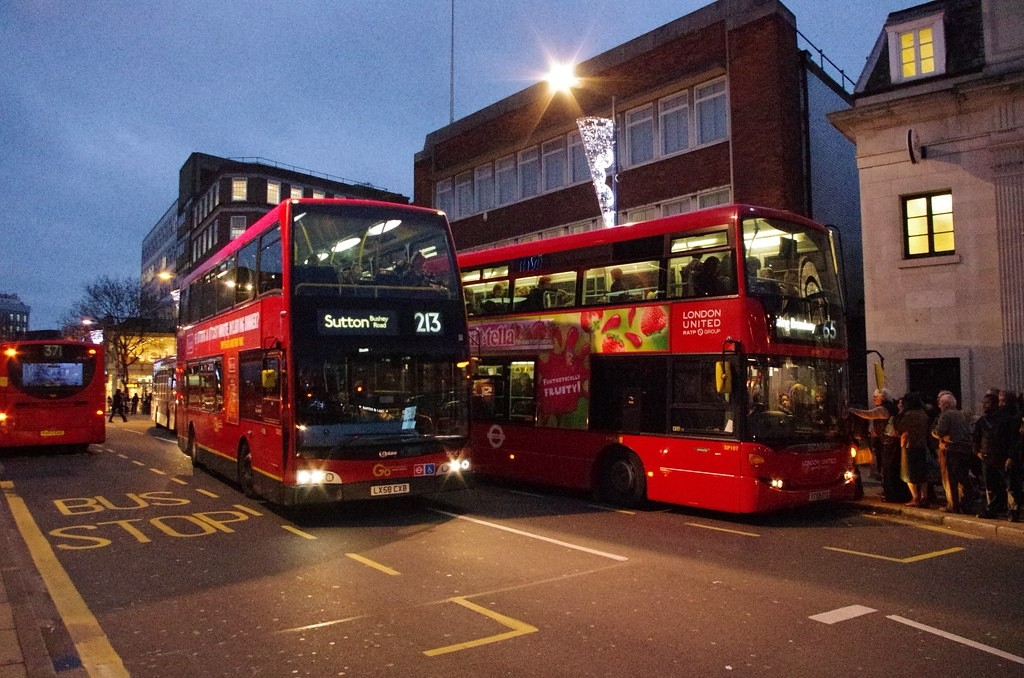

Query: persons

(145, 393), (152, 415)
(487, 284), (505, 299)
(108, 389), (129, 423)
(850, 388), (1024, 522)
(464, 288), (475, 313)
(131, 393), (139, 414)
(529, 277), (558, 310)
(512, 374), (533, 397)
(779, 383), (811, 414)
(609, 268), (626, 301)
(403, 252), (426, 275)
(123, 393), (130, 414)
(682, 246), (798, 298)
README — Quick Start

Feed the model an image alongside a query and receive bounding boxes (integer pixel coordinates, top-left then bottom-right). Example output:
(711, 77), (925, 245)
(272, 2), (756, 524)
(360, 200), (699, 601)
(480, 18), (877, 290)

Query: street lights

(551, 71), (617, 227)
(83, 319), (118, 396)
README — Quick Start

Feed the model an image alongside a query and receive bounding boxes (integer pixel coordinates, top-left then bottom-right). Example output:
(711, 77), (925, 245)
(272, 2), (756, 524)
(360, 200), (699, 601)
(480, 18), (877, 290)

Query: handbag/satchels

(854, 440), (874, 464)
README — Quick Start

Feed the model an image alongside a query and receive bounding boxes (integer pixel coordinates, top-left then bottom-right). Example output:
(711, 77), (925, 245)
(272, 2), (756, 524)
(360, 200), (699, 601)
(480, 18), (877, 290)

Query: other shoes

(977, 510), (998, 518)
(939, 504), (960, 514)
(108, 420), (114, 423)
(1006, 508), (1020, 522)
(123, 420), (128, 422)
(905, 497), (928, 508)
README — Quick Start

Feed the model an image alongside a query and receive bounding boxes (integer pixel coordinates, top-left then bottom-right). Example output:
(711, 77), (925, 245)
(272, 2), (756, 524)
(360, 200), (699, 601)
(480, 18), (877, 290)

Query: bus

(151, 356), (176, 434)
(175, 198), (481, 509)
(0, 340), (106, 450)
(457, 205), (887, 513)
